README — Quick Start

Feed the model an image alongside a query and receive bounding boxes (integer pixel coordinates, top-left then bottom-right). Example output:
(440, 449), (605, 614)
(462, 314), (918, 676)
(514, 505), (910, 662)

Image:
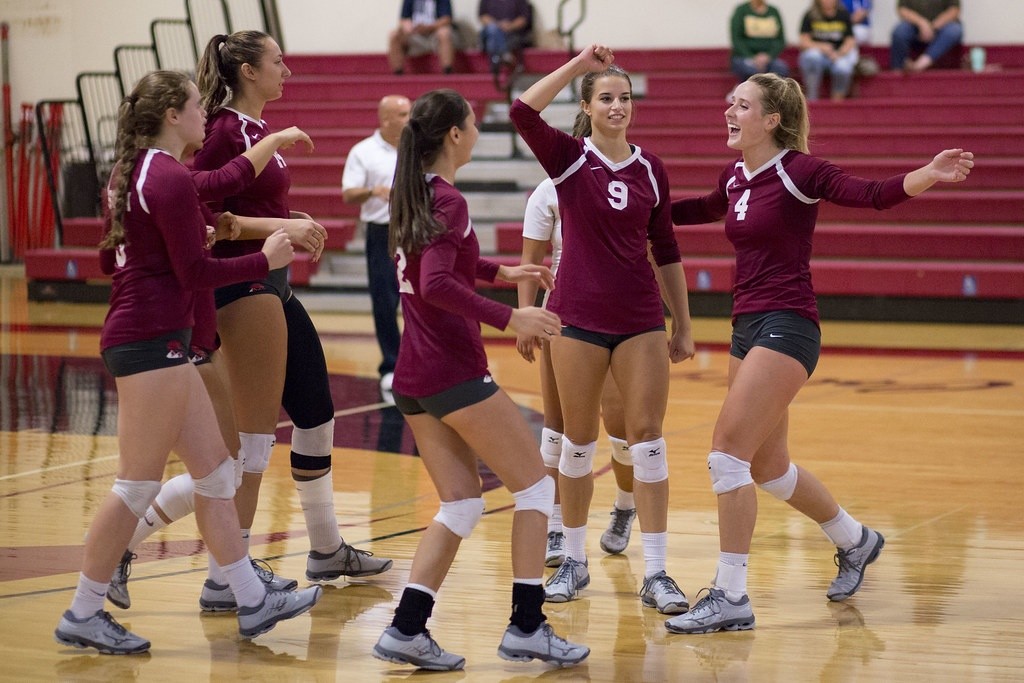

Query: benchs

(283, 43), (1024, 73)
(24, 73), (516, 305)
(472, 72), (1024, 325)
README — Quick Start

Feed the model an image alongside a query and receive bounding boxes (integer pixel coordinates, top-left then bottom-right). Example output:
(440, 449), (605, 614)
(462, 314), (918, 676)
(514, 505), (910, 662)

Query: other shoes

(380, 373), (393, 391)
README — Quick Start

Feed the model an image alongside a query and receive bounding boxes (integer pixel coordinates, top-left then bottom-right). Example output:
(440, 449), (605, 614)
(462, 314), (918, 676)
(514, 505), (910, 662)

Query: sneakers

(54, 648), (153, 683)
(250, 558), (298, 592)
(828, 526), (885, 602)
(496, 622), (591, 666)
(84, 531), (139, 609)
(308, 584), (392, 626)
(827, 601), (886, 665)
(236, 585), (322, 640)
(599, 504), (636, 554)
(305, 535), (393, 582)
(372, 627), (466, 671)
(666, 630), (756, 666)
(639, 571), (689, 613)
(545, 531), (566, 567)
(197, 579), (239, 611)
(54, 609), (151, 654)
(377, 667), (467, 682)
(666, 588), (756, 634)
(544, 555), (590, 602)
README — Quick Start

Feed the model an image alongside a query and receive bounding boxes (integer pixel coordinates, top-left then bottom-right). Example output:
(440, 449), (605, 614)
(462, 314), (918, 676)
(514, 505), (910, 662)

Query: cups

(969, 48), (986, 72)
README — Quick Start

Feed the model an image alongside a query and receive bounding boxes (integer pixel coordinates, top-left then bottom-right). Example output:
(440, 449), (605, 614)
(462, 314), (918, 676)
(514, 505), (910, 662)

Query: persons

(343, 96), (411, 372)
(508, 46), (694, 613)
(370, 90), (593, 669)
(517, 109), (638, 566)
(191, 29), (390, 610)
(797, 0), (860, 100)
(891, 0), (962, 73)
(389, 0), (460, 74)
(53, 70), (320, 652)
(839, 0), (872, 44)
(728, 0), (787, 78)
(479, 0), (533, 64)
(665, 74), (973, 634)
